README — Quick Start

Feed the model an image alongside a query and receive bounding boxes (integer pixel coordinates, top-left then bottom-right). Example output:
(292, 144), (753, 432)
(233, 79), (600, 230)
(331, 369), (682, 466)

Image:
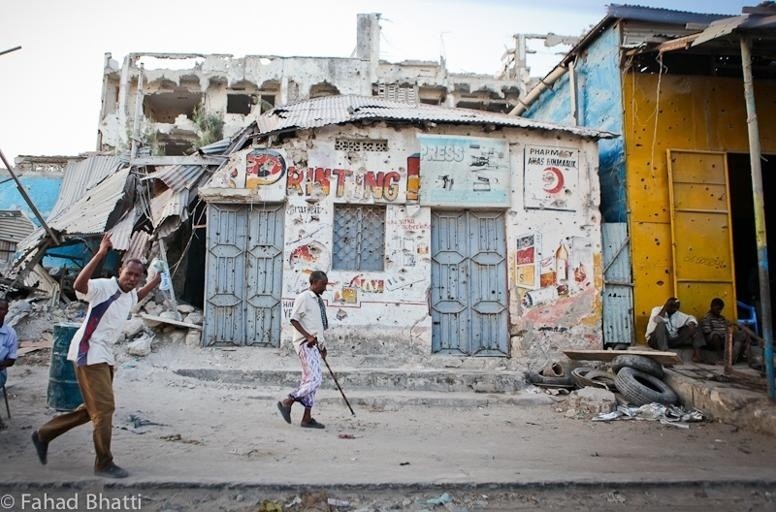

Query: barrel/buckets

(47, 321), (84, 413)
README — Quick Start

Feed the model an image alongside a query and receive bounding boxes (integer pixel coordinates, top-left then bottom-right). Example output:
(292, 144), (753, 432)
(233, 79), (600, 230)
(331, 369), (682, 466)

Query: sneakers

(93, 461), (128, 478)
(32, 431), (47, 465)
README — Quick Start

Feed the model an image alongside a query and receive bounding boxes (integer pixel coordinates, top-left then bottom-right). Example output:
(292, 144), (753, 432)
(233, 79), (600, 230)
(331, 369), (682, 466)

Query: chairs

(736, 298), (758, 333)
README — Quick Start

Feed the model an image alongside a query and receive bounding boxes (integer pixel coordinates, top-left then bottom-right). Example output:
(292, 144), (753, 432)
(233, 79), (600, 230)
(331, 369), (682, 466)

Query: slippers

(276, 400), (291, 424)
(300, 418), (325, 428)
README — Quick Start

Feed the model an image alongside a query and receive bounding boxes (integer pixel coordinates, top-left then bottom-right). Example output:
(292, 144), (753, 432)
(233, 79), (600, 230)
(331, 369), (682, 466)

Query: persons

(700, 298), (751, 366)
(646, 297), (706, 365)
(277, 270), (330, 430)
(0, 298), (19, 393)
(32, 231), (162, 479)
